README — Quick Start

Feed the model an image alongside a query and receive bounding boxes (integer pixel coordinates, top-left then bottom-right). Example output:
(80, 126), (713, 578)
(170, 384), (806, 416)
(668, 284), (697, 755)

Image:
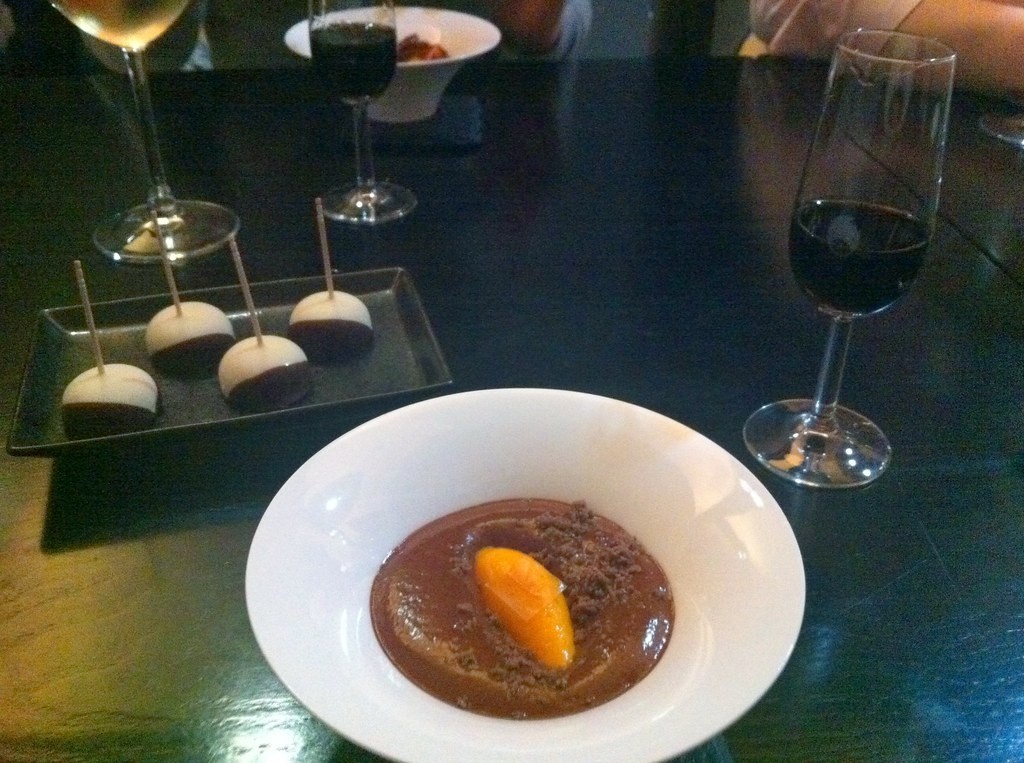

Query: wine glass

(307, 0), (417, 222)
(744, 28), (955, 488)
(52, 0), (241, 263)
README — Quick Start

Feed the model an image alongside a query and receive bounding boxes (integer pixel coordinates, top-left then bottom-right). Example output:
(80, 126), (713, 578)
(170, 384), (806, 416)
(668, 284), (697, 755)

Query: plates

(1, 265), (453, 455)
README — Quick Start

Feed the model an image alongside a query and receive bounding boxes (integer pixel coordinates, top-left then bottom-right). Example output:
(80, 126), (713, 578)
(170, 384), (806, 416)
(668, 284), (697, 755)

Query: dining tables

(0, 48), (1024, 763)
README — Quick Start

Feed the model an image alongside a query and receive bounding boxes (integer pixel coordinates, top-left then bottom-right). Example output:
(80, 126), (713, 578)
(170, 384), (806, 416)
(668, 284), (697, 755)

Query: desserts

(62, 290), (374, 439)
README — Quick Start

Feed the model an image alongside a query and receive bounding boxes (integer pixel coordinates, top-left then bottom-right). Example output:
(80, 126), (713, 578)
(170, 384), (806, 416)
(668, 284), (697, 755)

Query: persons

(490, 0), (592, 61)
(748, 0), (1024, 94)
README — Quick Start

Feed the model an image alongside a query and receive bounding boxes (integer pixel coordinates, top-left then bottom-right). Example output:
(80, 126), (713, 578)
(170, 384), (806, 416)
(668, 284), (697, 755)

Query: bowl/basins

(285, 7), (502, 121)
(246, 388), (804, 763)
